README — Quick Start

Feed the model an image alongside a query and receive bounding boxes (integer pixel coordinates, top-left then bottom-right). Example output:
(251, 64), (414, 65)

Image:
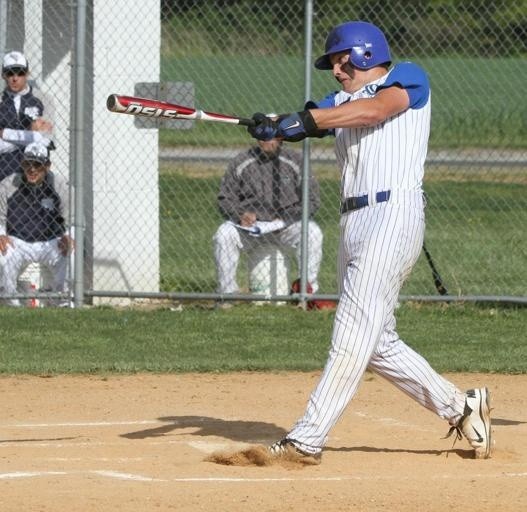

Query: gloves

(246, 111), (278, 141)
(275, 109), (324, 142)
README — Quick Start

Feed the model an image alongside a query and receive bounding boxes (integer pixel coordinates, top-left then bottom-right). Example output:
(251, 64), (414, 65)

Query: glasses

(21, 161), (41, 169)
(4, 68), (25, 78)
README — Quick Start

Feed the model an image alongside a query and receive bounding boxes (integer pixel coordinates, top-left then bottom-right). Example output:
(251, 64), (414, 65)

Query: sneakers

(441, 385), (493, 461)
(263, 438), (325, 466)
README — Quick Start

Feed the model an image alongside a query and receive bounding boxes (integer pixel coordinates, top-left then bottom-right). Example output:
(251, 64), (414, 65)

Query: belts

(339, 190), (391, 215)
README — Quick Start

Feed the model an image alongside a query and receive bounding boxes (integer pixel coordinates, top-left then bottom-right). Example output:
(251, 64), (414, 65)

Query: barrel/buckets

(247, 244), (290, 304)
(19, 262), (52, 307)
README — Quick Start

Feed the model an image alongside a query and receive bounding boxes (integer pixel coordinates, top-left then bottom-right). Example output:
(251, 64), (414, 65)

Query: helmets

(314, 20), (393, 71)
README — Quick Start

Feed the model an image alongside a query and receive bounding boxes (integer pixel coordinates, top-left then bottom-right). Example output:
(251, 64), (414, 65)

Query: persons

(245, 20), (494, 470)
(210, 111), (326, 311)
(0, 143), (75, 312)
(0, 48), (60, 184)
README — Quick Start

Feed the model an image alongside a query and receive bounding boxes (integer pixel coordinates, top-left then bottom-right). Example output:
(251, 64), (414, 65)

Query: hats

(17, 141), (52, 165)
(3, 49), (28, 70)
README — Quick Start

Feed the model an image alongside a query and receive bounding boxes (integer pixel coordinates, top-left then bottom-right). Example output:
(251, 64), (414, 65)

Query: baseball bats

(106, 94), (257, 129)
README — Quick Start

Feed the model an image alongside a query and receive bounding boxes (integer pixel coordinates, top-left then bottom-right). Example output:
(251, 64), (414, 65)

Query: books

(225, 213), (285, 238)
